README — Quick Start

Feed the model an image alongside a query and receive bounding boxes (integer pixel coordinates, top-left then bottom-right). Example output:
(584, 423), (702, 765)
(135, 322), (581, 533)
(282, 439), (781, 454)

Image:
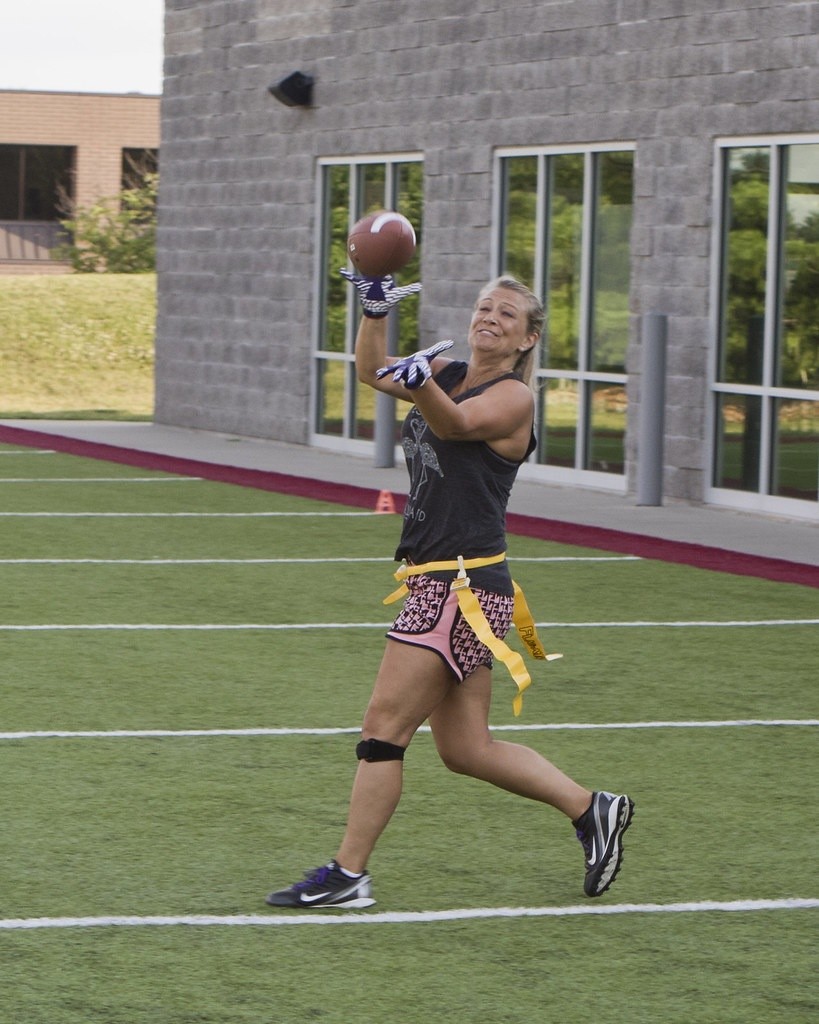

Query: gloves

(342, 267), (421, 318)
(376, 339), (455, 389)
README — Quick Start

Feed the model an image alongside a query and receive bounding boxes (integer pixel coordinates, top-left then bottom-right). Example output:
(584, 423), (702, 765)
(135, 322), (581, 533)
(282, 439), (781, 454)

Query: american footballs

(345, 208), (418, 279)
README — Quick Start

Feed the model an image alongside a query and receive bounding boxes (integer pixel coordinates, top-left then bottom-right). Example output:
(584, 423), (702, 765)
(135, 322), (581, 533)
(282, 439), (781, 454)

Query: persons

(268, 263), (633, 913)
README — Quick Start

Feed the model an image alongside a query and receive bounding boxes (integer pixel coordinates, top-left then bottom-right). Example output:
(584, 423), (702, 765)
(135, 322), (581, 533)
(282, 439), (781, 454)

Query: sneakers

(266, 864), (377, 909)
(572, 791), (639, 897)
